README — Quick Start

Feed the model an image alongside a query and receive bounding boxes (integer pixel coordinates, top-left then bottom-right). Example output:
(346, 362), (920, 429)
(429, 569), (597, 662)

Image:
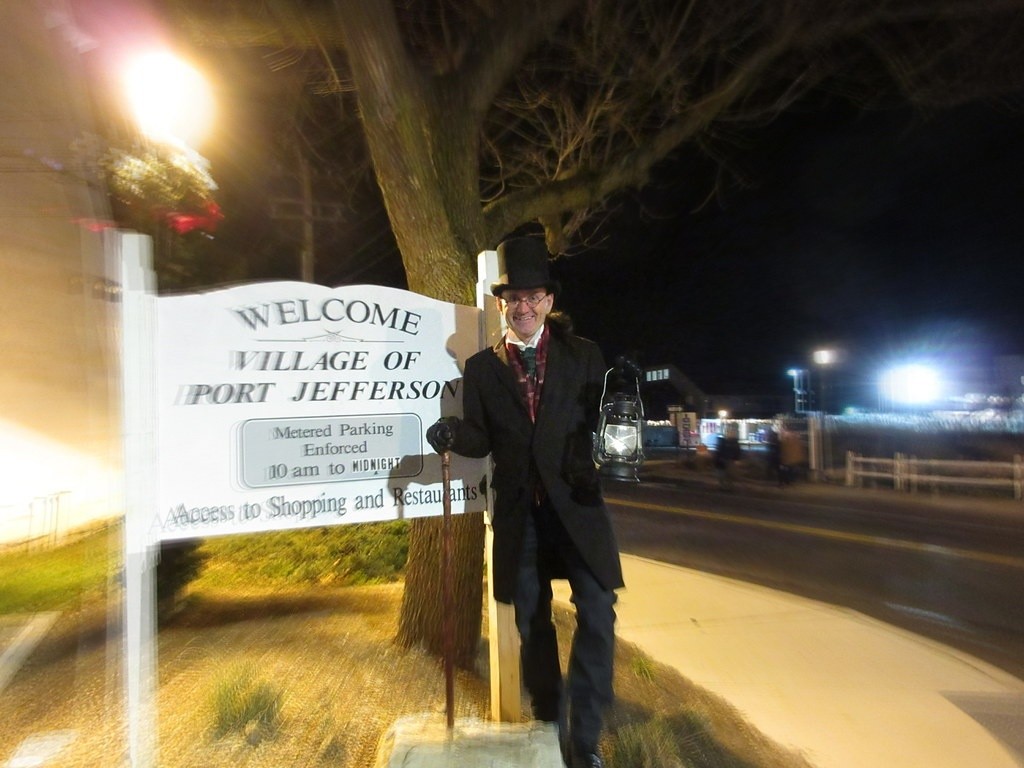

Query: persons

(425, 236), (645, 768)
(714, 427), (743, 488)
(772, 431), (808, 484)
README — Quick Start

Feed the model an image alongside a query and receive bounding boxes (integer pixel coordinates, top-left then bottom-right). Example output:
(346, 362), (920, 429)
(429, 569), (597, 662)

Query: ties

(521, 347), (536, 382)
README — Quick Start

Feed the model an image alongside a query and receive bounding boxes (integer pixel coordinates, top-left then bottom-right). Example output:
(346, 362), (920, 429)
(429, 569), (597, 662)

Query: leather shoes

(570, 739), (602, 768)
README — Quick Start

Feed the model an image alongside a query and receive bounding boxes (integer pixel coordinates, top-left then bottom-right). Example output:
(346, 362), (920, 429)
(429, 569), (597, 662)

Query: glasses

(501, 294), (546, 308)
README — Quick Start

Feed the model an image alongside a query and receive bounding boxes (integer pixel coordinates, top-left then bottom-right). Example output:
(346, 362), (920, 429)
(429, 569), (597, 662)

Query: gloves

(426, 417), (460, 456)
(610, 355), (643, 386)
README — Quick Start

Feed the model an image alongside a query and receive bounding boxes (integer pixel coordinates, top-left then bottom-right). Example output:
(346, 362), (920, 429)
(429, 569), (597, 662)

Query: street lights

(812, 350), (833, 470)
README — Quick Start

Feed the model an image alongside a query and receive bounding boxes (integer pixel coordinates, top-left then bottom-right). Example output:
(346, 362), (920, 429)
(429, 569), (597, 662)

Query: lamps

(594, 367), (646, 483)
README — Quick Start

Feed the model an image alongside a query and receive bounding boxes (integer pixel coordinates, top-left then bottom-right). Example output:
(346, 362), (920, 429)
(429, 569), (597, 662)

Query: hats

(491, 238), (562, 298)
